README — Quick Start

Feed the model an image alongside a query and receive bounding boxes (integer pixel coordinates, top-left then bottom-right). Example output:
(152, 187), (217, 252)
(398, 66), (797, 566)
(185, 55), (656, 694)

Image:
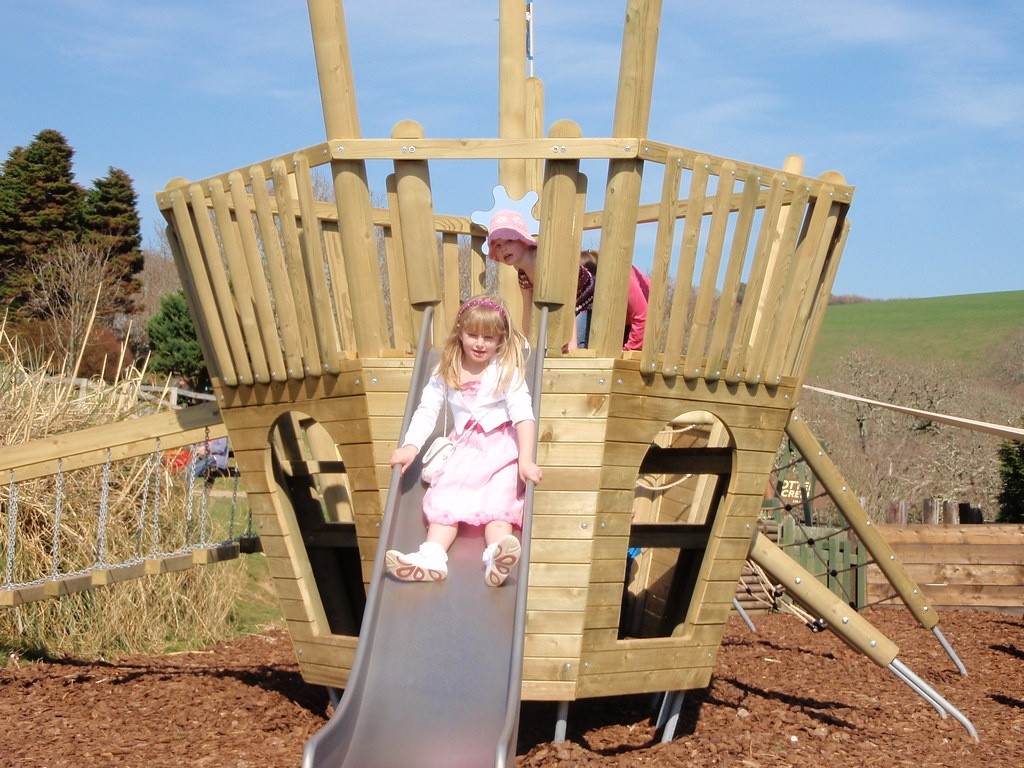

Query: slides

(302, 305), (545, 768)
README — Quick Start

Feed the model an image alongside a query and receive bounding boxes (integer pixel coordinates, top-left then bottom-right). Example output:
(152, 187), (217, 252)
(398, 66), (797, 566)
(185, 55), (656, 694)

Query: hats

(487, 210), (538, 262)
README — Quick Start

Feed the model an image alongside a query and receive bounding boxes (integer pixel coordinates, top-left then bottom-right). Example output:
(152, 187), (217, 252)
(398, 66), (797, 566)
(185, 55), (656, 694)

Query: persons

(185, 436), (228, 479)
(382, 294), (541, 587)
(469, 184), (650, 352)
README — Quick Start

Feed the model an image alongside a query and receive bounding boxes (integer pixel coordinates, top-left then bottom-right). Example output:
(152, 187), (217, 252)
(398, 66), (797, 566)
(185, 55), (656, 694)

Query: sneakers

(485, 535), (522, 587)
(385, 543), (448, 582)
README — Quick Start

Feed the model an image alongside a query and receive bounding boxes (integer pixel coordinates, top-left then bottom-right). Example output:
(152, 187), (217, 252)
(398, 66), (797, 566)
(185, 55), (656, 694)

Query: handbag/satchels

(421, 436), (455, 485)
